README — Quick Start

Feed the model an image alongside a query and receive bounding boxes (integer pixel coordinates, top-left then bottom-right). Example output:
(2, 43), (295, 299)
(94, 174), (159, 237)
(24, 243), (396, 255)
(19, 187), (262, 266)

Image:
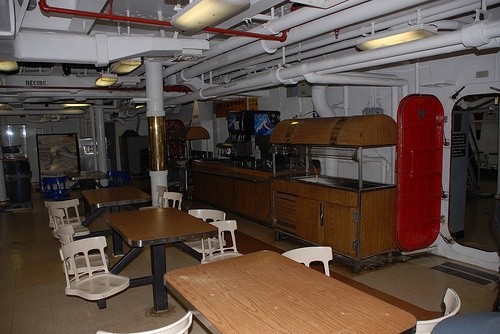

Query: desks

(98, 207), (204, 312)
(63, 169), (108, 217)
(163, 251), (417, 334)
(74, 184), (151, 257)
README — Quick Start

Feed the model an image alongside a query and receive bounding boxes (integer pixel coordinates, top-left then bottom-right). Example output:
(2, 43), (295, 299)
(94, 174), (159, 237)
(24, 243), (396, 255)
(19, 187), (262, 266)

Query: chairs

(96, 311), (193, 334)
(201, 220), (243, 264)
(415, 288), (461, 334)
(282, 246), (333, 277)
(41, 176), (130, 301)
(108, 170), (128, 186)
(183, 209), (227, 253)
(138, 192), (182, 211)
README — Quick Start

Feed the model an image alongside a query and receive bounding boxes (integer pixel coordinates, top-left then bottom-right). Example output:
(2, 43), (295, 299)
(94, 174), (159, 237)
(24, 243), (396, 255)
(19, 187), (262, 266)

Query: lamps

(169, 0), (252, 36)
(111, 58), (142, 75)
(354, 23), (438, 50)
(95, 67), (118, 87)
